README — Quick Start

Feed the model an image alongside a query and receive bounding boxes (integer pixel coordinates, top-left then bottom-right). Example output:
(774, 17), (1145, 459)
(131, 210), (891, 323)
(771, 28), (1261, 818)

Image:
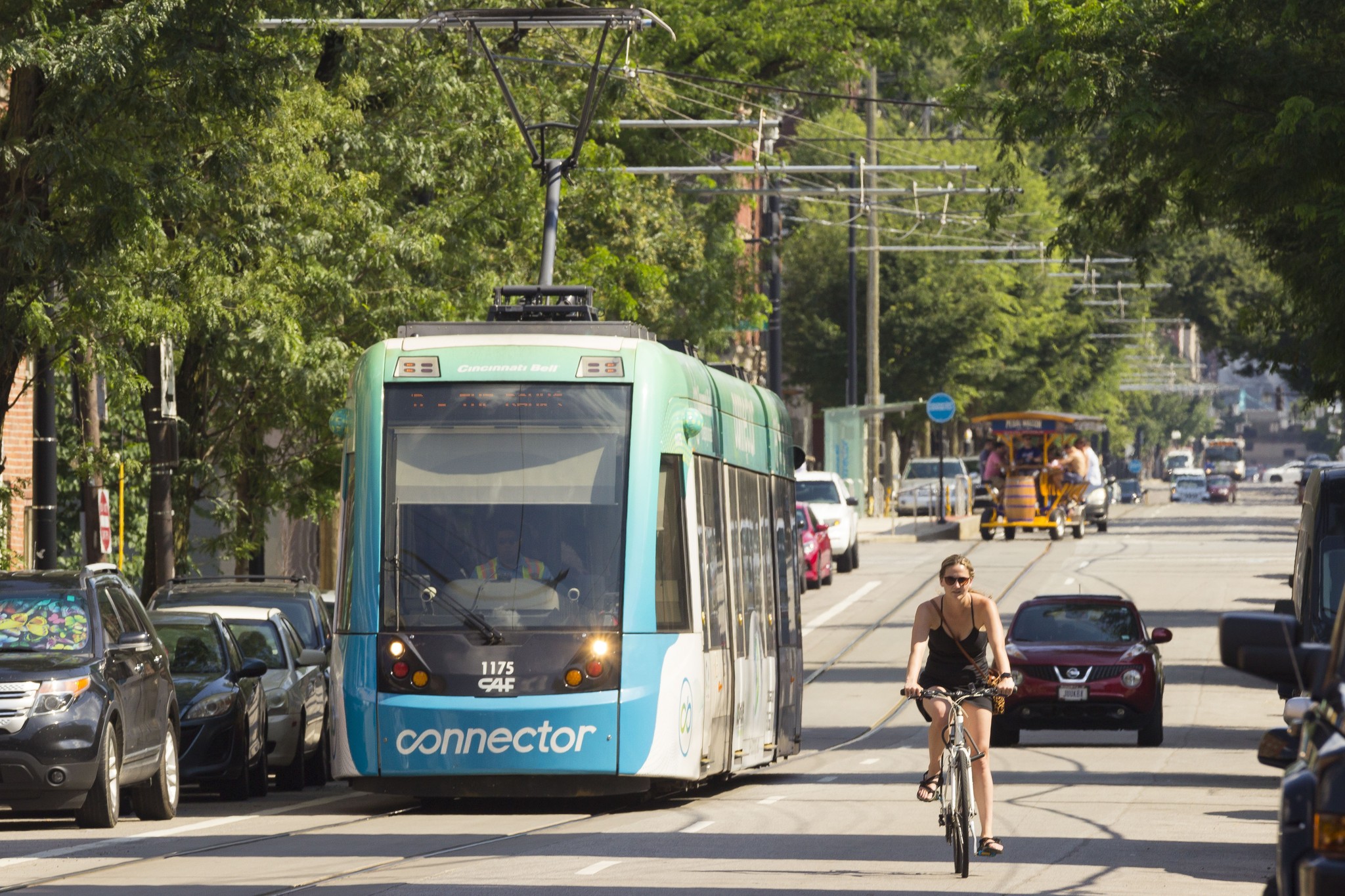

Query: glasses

(944, 575), (971, 586)
(497, 537), (520, 544)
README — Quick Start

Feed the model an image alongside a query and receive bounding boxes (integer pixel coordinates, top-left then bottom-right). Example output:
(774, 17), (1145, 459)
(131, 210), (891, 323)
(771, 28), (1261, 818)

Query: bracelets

(1001, 673), (1014, 681)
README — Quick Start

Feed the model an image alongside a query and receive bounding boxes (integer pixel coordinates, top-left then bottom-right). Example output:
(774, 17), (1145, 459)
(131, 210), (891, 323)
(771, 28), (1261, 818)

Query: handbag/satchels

(987, 667), (1006, 716)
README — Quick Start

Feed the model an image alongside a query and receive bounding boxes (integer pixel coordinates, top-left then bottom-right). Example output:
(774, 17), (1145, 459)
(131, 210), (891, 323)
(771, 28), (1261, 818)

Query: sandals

(917, 769), (944, 802)
(977, 837), (1003, 854)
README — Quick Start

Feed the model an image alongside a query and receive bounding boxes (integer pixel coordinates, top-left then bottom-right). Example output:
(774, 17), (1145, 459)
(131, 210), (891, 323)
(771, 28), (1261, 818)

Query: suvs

(145, 574), (332, 663)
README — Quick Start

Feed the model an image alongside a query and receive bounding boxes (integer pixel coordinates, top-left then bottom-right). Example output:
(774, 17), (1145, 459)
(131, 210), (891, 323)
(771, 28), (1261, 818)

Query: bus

(329, 332), (807, 800)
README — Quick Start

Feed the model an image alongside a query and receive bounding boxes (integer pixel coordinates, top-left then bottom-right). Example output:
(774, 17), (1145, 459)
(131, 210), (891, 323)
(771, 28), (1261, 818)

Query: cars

(319, 592), (336, 630)
(895, 456), (978, 517)
(1020, 462), (1117, 533)
(795, 501), (833, 590)
(794, 470), (860, 573)
(142, 611), (269, 800)
(156, 603), (328, 789)
(961, 456), (999, 515)
(991, 593), (1173, 749)
(1161, 431), (1344, 896)
(0, 562), (184, 829)
(1117, 479), (1151, 504)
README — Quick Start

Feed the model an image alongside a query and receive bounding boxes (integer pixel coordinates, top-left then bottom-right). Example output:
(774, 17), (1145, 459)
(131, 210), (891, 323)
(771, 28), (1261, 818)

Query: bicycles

(898, 680), (1015, 878)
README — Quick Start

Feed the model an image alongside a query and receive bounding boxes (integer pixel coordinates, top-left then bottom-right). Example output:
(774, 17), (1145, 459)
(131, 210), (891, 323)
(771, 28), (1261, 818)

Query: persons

(904, 555), (1015, 853)
(472, 524), (556, 588)
(980, 433), (1105, 517)
